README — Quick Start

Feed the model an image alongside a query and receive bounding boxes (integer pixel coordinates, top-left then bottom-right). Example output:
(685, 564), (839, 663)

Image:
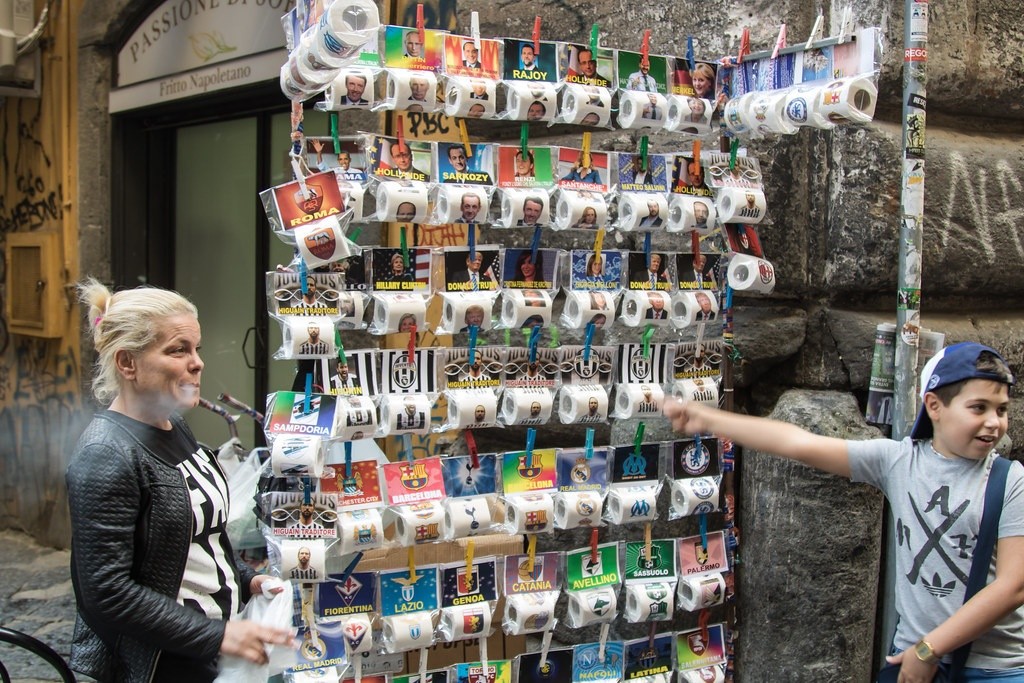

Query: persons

(662, 342), (1024, 683)
(67, 272), (299, 683)
(275, 30), (764, 683)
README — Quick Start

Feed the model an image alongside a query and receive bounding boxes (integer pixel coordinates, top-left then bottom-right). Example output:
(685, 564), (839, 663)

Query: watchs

(914, 636), (943, 665)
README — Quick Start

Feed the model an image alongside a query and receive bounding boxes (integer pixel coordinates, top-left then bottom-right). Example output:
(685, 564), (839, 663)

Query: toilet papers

(235, 0), (881, 683)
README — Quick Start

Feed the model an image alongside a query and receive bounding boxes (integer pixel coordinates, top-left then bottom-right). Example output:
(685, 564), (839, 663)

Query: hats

(912, 342), (1013, 440)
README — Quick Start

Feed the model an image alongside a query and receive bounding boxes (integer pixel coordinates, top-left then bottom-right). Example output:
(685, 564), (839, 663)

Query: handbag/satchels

(214, 579), (300, 682)
